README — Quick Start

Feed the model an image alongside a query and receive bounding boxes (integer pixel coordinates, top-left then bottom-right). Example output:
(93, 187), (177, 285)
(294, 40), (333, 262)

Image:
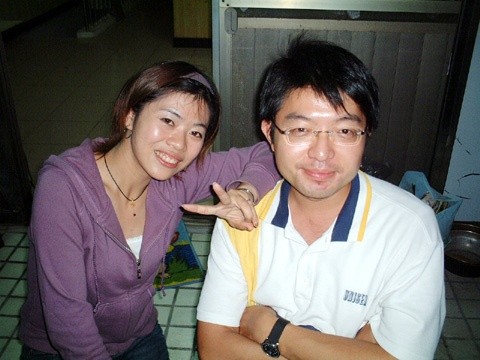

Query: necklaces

(100, 149), (150, 217)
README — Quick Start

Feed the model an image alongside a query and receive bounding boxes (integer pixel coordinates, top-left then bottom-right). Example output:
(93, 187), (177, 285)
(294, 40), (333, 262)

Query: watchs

(261, 316), (290, 358)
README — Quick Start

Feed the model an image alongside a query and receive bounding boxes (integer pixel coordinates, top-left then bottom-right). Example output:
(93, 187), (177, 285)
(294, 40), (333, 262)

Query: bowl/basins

(441, 222), (480, 277)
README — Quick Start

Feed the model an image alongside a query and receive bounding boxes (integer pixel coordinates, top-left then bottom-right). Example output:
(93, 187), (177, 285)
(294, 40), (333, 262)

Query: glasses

(272, 119), (374, 147)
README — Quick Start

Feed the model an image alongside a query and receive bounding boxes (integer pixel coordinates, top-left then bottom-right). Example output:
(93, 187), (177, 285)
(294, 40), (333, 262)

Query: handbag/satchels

(399, 170), (464, 247)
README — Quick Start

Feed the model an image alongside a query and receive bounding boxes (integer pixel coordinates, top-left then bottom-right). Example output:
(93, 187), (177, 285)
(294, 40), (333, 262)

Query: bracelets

(235, 187), (255, 205)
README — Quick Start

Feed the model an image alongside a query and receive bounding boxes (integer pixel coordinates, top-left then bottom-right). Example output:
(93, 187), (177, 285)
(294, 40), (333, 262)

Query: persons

(16, 61), (284, 360)
(195, 29), (449, 360)
